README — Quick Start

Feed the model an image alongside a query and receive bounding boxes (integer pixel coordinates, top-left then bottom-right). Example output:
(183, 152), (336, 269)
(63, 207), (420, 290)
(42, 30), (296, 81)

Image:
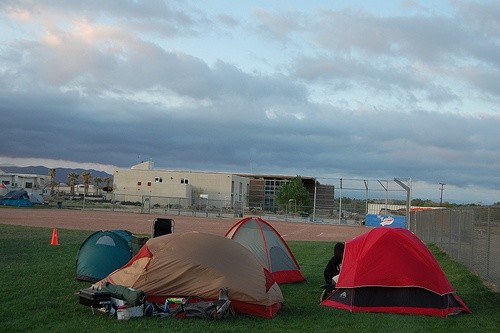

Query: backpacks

(183, 296), (235, 320)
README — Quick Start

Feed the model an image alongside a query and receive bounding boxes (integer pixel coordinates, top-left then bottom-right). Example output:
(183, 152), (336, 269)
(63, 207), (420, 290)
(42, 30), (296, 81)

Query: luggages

(79, 288), (112, 308)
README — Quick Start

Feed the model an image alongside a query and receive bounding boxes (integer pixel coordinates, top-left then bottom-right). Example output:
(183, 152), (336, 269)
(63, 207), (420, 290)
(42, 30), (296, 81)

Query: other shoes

(320, 289), (326, 302)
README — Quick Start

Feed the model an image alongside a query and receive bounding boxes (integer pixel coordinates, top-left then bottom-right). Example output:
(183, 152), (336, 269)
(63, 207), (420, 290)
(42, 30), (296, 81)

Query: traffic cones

(49, 227), (60, 245)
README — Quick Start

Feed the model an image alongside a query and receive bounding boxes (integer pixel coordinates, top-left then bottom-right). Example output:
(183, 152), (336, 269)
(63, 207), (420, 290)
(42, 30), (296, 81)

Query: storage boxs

(78, 288), (111, 308)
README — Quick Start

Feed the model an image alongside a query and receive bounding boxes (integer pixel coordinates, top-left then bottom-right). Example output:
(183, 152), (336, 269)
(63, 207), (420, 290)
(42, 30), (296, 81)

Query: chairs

(151, 217), (175, 238)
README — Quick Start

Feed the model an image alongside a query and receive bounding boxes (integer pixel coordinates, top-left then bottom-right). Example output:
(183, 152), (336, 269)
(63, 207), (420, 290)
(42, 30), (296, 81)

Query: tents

(224, 216), (306, 285)
(88, 231), (285, 320)
(320, 227), (472, 318)
(76, 229), (132, 283)
